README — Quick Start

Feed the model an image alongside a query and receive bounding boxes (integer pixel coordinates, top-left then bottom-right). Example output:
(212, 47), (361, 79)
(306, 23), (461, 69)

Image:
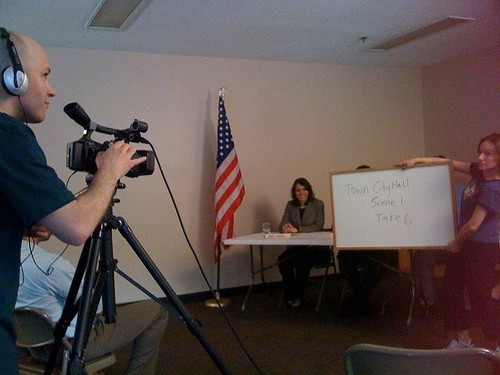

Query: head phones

(0, 26), (28, 95)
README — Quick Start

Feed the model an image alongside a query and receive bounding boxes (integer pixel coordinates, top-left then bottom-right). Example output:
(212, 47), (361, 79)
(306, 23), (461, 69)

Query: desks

(222, 231), (333, 317)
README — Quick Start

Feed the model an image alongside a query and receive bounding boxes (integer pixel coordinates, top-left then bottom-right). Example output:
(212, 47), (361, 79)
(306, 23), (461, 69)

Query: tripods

(43, 177), (233, 375)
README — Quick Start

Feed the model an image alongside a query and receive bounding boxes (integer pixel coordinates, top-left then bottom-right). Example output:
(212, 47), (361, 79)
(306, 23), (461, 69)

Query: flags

(214, 97), (246, 263)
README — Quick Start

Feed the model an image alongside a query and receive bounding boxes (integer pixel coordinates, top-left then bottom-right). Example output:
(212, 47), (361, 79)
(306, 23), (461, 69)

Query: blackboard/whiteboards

(329, 160), (458, 250)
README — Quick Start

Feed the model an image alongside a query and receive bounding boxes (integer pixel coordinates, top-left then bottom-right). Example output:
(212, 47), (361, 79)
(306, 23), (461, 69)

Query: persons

(337, 165), (384, 306)
(278, 177), (325, 313)
(15, 232), (170, 375)
(394, 133), (500, 350)
(0, 27), (147, 375)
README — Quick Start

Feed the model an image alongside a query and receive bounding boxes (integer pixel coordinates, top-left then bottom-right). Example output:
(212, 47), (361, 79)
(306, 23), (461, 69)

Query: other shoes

(443, 340), (473, 351)
(471, 340), (499, 352)
(285, 298), (302, 312)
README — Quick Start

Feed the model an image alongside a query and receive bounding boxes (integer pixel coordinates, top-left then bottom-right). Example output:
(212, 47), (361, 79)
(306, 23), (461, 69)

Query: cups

(261, 222), (270, 240)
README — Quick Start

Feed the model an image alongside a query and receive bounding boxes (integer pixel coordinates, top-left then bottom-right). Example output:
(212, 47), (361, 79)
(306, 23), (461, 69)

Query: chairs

(278, 226), (342, 311)
(15, 307), (116, 375)
(342, 344), (500, 375)
